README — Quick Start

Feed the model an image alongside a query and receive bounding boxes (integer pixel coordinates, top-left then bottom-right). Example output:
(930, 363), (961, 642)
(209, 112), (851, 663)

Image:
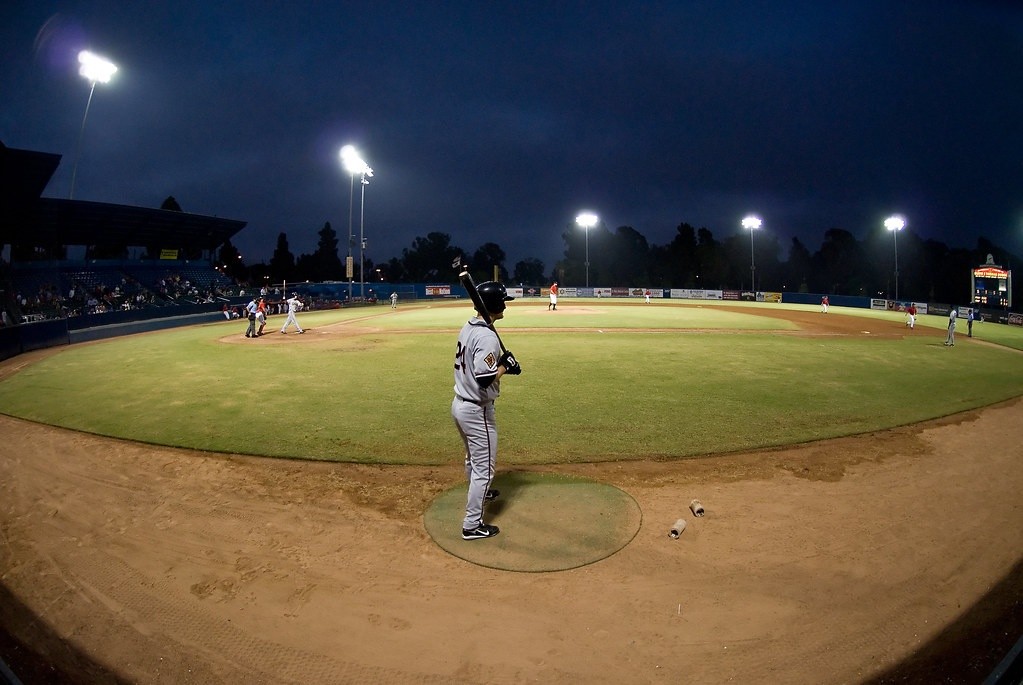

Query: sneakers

(484, 489), (500, 501)
(462, 524), (499, 539)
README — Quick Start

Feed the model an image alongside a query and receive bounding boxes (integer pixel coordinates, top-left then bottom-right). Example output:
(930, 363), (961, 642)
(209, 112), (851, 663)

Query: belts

(463, 398), (478, 404)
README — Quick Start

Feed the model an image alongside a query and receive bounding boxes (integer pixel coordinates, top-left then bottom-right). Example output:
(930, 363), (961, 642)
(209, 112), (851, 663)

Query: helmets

(260, 295), (265, 300)
(291, 292), (298, 297)
(253, 297), (258, 303)
(474, 281), (514, 315)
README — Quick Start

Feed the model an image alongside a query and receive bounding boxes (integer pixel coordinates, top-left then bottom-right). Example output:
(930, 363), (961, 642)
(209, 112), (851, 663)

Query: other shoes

(281, 330), (287, 334)
(299, 332), (304, 334)
(245, 334), (250, 338)
(252, 334), (258, 338)
(257, 332), (264, 336)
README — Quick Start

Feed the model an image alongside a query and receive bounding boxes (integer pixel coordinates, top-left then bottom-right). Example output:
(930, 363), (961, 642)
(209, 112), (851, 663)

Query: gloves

(496, 350), (517, 373)
(503, 362), (521, 375)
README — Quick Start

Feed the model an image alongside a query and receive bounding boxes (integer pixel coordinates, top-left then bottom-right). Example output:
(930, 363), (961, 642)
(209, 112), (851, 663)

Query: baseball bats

(459, 271), (522, 375)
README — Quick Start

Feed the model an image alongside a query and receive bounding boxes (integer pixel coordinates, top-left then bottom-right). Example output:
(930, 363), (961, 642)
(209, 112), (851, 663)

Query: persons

(645, 289), (651, 304)
(255, 298), (268, 335)
(245, 297), (259, 338)
(2, 308), (7, 322)
(778, 296), (781, 303)
(905, 303), (917, 329)
(597, 291), (601, 298)
(979, 313), (985, 322)
(966, 309), (974, 336)
(391, 292), (398, 308)
(10, 272), (280, 318)
(451, 281), (520, 539)
(549, 281), (558, 310)
(223, 303), (230, 320)
(279, 292), (304, 334)
(232, 306), (240, 318)
(944, 305), (957, 347)
(821, 296), (829, 313)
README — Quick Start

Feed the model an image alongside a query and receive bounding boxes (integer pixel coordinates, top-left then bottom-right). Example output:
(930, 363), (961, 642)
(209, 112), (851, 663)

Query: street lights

(576, 215), (597, 286)
(358, 160), (374, 305)
(741, 217), (762, 291)
(338, 144), (364, 305)
(884, 217), (904, 299)
(68, 51), (118, 202)
